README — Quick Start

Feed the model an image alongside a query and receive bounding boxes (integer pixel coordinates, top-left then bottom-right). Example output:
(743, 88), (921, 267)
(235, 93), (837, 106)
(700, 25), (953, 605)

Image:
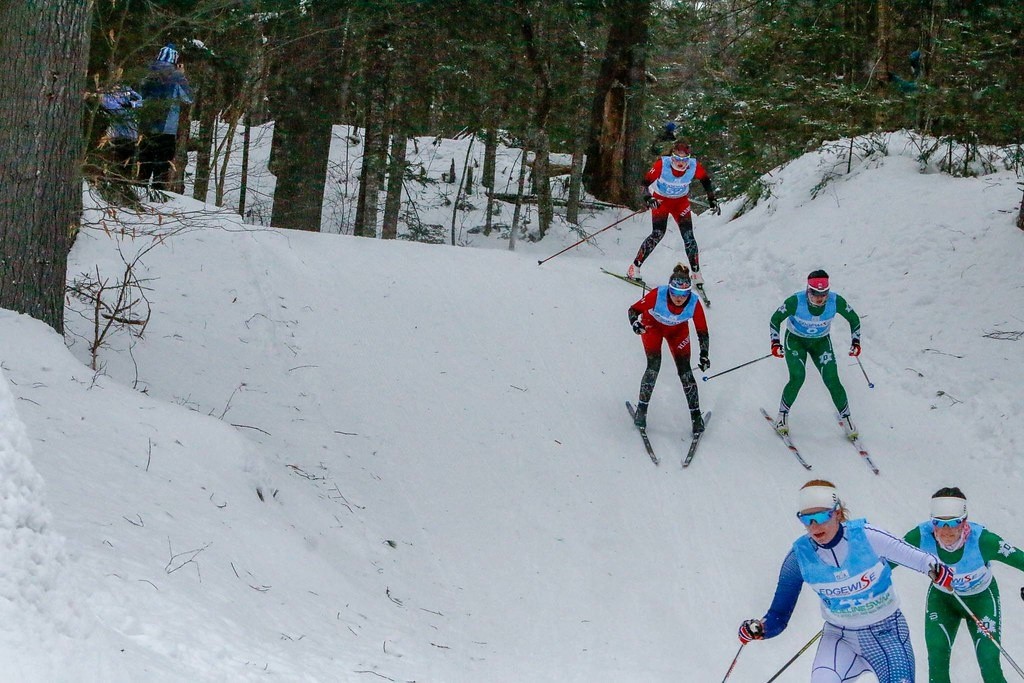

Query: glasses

(796, 501), (838, 527)
(671, 152), (691, 163)
(669, 286), (690, 296)
(810, 289), (829, 298)
(929, 513), (967, 529)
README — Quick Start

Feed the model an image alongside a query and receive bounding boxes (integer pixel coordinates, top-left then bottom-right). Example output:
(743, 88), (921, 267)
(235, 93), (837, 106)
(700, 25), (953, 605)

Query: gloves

(631, 318), (646, 335)
(738, 618), (765, 646)
(709, 199), (720, 216)
(698, 350), (710, 372)
(645, 196), (658, 209)
(770, 339), (784, 358)
(927, 563), (955, 593)
(849, 339), (860, 356)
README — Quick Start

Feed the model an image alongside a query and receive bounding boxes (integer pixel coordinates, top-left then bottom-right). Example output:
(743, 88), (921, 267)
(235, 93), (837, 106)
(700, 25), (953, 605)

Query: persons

(738, 480), (953, 683)
(84, 69), (142, 178)
(649, 122), (676, 156)
(140, 44), (195, 189)
(628, 265), (711, 433)
(626, 142), (721, 285)
(770, 270), (860, 437)
(885, 487), (1024, 683)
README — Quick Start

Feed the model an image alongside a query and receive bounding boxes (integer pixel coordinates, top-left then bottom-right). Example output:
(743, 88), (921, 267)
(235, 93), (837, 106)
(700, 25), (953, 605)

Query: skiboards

(759, 407), (880, 483)
(625, 400), (711, 467)
(81, 161), (147, 214)
(598, 267), (711, 308)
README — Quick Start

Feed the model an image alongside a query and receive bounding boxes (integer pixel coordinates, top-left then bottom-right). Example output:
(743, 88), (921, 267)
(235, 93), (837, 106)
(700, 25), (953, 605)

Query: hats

(668, 263), (691, 291)
(664, 122), (677, 131)
(157, 43), (179, 64)
(807, 269), (829, 293)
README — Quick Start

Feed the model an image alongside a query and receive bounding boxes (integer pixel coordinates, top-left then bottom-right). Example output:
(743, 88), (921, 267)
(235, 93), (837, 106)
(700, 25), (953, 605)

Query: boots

(634, 400), (648, 431)
(690, 408), (704, 433)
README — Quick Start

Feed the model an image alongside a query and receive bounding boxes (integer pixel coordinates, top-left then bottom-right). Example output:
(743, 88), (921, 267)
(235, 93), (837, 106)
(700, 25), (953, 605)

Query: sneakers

(691, 270), (704, 285)
(776, 410), (789, 436)
(843, 417), (859, 440)
(626, 264), (642, 280)
